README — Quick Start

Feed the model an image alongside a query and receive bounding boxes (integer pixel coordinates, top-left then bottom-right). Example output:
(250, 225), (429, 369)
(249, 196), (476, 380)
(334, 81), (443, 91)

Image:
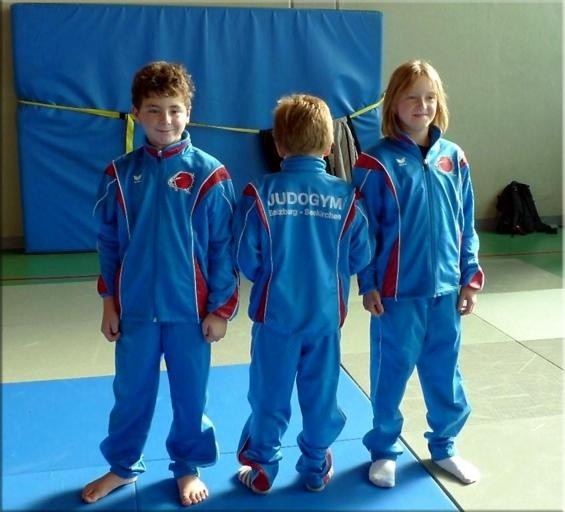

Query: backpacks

(493, 180), (542, 238)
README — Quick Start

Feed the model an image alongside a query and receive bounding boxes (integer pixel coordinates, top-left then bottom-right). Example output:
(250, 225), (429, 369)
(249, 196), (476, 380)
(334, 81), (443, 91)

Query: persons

(230, 92), (376, 495)
(81, 61), (239, 507)
(351, 59), (486, 490)
